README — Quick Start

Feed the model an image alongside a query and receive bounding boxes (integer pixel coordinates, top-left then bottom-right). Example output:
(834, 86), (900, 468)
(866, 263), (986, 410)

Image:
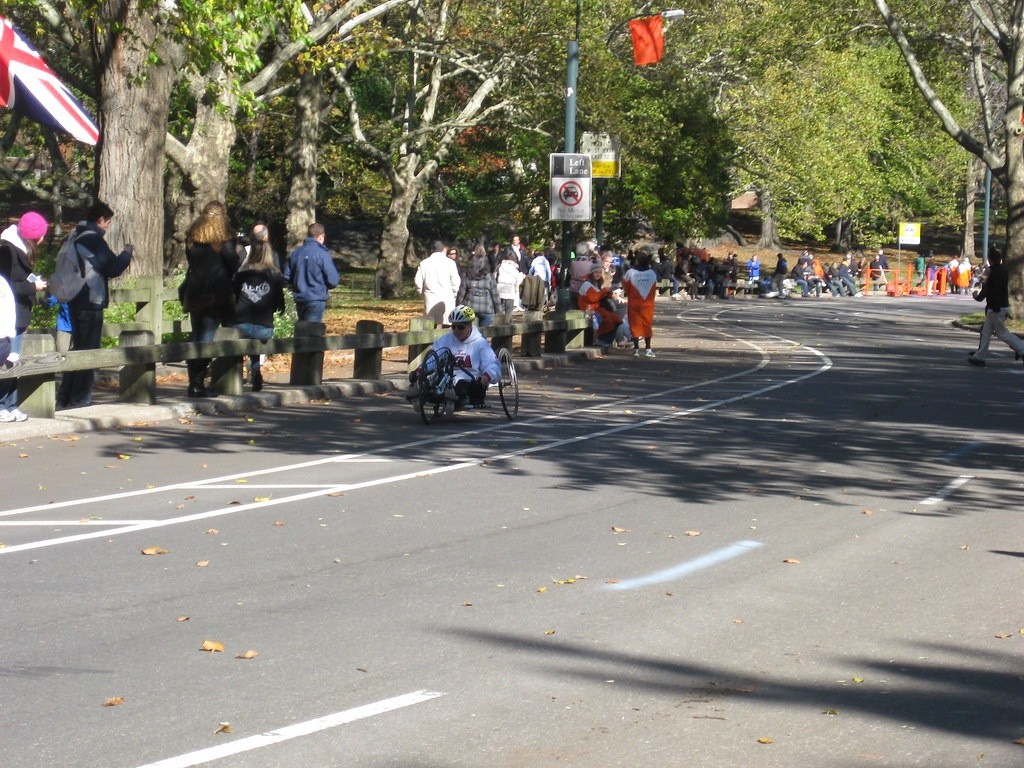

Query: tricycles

(408, 346), (520, 426)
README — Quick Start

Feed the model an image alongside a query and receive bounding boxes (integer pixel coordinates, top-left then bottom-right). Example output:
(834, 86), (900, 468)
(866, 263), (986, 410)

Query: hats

(590, 264), (601, 273)
(17, 211), (47, 238)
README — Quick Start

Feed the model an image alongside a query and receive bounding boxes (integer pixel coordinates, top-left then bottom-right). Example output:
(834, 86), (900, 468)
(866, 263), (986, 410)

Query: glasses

(452, 323), (469, 330)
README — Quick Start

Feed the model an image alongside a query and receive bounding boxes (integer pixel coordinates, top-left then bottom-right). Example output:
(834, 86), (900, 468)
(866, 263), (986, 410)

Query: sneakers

(0, 410), (15, 421)
(10, 409), (27, 421)
(645, 349), (656, 357)
(633, 349), (640, 356)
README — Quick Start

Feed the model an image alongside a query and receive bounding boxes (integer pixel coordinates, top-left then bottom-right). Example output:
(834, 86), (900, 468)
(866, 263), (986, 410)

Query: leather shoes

(188, 383), (220, 397)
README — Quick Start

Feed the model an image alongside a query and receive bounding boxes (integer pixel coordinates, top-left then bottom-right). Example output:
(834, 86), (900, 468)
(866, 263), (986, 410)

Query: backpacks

(51, 228), (97, 301)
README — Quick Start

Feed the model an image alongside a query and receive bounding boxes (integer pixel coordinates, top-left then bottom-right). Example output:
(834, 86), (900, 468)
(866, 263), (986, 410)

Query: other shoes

(408, 384), (420, 411)
(252, 371), (262, 392)
(968, 356), (985, 367)
(444, 388), (455, 416)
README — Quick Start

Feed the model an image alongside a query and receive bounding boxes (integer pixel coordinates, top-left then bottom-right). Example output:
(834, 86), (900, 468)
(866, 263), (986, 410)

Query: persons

(415, 234), (890, 357)
(283, 223), (339, 322)
(65, 203), (134, 410)
(409, 305), (502, 417)
(183, 200), (286, 398)
(0, 212), (87, 422)
(915, 249), (1024, 365)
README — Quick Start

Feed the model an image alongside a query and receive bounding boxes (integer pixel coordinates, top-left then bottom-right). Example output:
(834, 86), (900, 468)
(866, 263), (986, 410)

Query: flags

(629, 15), (663, 65)
(0, 13), (100, 146)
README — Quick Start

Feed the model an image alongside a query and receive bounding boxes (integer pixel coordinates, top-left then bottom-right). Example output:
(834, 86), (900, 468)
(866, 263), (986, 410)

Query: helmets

(448, 305), (475, 322)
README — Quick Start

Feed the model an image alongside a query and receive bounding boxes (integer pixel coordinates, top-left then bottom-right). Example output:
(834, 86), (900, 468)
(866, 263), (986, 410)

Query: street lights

(596, 6), (687, 250)
